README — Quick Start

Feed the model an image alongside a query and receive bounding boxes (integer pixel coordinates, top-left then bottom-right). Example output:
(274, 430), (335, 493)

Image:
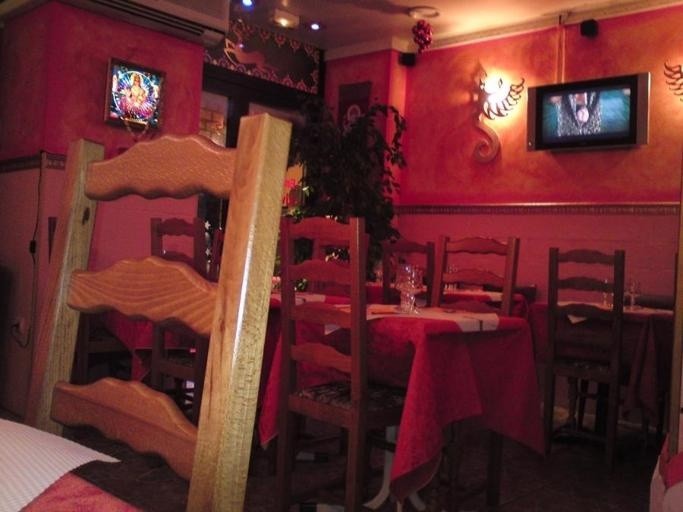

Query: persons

(571, 102), (592, 128)
(120, 73), (148, 116)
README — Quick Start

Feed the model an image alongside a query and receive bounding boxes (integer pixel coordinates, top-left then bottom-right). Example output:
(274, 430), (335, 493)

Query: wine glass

(400, 270), (423, 317)
(626, 280), (641, 312)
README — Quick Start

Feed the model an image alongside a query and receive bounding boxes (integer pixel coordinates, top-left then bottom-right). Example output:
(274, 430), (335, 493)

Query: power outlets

(9, 315), (32, 346)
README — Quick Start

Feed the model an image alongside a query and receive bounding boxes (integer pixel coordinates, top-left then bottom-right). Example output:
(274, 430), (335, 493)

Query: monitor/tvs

(525, 71), (651, 153)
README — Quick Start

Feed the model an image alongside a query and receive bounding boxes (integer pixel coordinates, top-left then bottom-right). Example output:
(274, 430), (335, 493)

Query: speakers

(579, 20), (598, 38)
(398, 51), (417, 65)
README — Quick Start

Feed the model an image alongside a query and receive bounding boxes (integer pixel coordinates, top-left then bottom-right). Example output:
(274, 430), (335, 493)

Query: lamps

(464, 58), (526, 169)
